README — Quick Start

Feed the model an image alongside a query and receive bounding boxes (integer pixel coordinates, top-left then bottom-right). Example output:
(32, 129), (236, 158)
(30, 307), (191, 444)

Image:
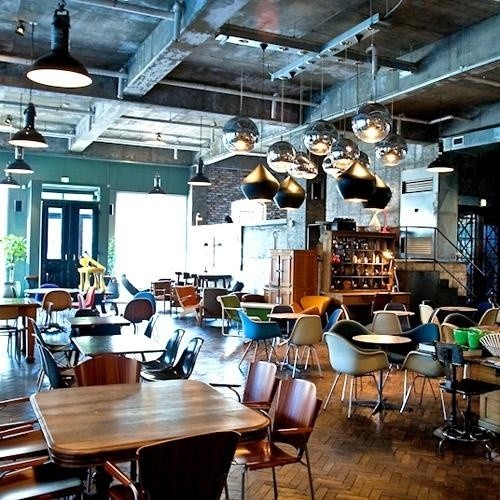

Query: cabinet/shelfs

(323, 231), (397, 292)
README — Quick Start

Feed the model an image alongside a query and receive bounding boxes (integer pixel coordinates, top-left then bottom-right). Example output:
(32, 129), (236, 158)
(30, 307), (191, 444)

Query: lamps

(428, 122), (453, 174)
(0, 125), (21, 189)
(9, 22), (48, 148)
(5, 93), (33, 173)
(147, 134), (166, 195)
(216, 16), (417, 210)
(26, 0), (92, 87)
(188, 115), (211, 187)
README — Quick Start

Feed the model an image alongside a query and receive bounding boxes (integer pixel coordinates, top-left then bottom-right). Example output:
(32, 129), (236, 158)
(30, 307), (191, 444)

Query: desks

(30, 380), (270, 494)
(71, 316), (129, 334)
(241, 301), (275, 321)
(374, 310), (414, 317)
(461, 354), (499, 434)
(267, 312), (319, 372)
(72, 334), (166, 357)
(0, 297), (41, 363)
(25, 276), (37, 296)
(321, 293), (411, 308)
(441, 307), (477, 311)
(352, 335), (413, 415)
(25, 288), (105, 313)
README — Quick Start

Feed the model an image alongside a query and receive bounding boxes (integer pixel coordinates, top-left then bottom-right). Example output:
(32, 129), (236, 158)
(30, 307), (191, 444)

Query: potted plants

(0, 234), (27, 297)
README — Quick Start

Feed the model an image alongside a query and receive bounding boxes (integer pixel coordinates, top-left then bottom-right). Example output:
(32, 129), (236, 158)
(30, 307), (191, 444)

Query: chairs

(434, 343), (499, 461)
(200, 289), (228, 327)
(106, 278), (119, 315)
(72, 309), (99, 316)
(40, 346), (73, 390)
(105, 430), (241, 500)
(176, 286), (199, 321)
(170, 286), (181, 314)
(73, 354), (142, 384)
(135, 292), (156, 312)
(341, 304), (351, 320)
(36, 284), (58, 302)
(140, 337), (203, 382)
(268, 305), (301, 365)
(124, 299), (152, 334)
(371, 294), (391, 315)
(217, 294), (261, 338)
(281, 317), (324, 378)
(488, 298), (500, 308)
(293, 296), (330, 314)
(78, 294), (86, 309)
(478, 308), (499, 325)
(32, 334), (75, 390)
(42, 291), (72, 325)
(236, 309), (282, 369)
(232, 282), (244, 291)
(443, 313), (476, 328)
(423, 300), (430, 304)
(72, 286), (95, 308)
(385, 303), (409, 328)
(0, 397), (37, 428)
(243, 294), (264, 301)
(30, 318), (73, 366)
(210, 360), (281, 440)
(383, 324), (439, 402)
(0, 324), (17, 358)
(399, 344), (444, 415)
(11, 286), (28, 329)
(0, 425), (48, 471)
(330, 320), (373, 396)
(138, 328), (184, 370)
(322, 332), (390, 419)
(419, 304), (440, 328)
(439, 323), (459, 343)
(123, 312), (158, 361)
(270, 249), (317, 305)
(372, 313), (402, 335)
(224, 379), (323, 500)
(317, 308), (342, 345)
(472, 303), (492, 318)
(122, 275), (149, 295)
(0, 462), (84, 500)
(151, 282), (171, 314)
